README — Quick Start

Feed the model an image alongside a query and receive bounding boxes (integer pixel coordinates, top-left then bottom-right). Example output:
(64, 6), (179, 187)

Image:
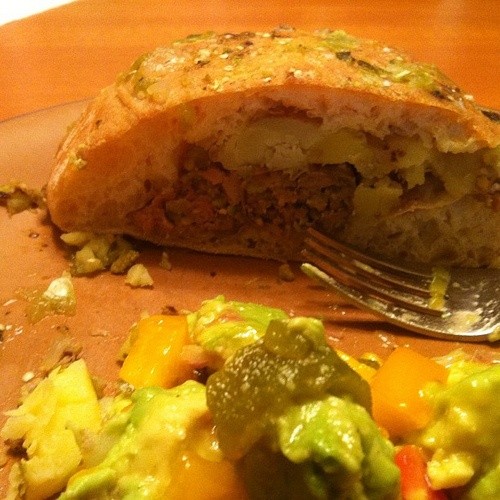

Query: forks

(302, 227), (500, 341)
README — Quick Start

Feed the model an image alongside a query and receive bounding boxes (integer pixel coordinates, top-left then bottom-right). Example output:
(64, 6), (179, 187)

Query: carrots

(117, 313), (451, 500)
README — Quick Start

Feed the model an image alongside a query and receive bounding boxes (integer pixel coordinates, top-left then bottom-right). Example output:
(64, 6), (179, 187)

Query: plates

(0, 96), (500, 499)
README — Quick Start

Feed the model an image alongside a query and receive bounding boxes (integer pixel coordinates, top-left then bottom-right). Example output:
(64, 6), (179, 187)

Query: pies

(45, 26), (500, 275)
